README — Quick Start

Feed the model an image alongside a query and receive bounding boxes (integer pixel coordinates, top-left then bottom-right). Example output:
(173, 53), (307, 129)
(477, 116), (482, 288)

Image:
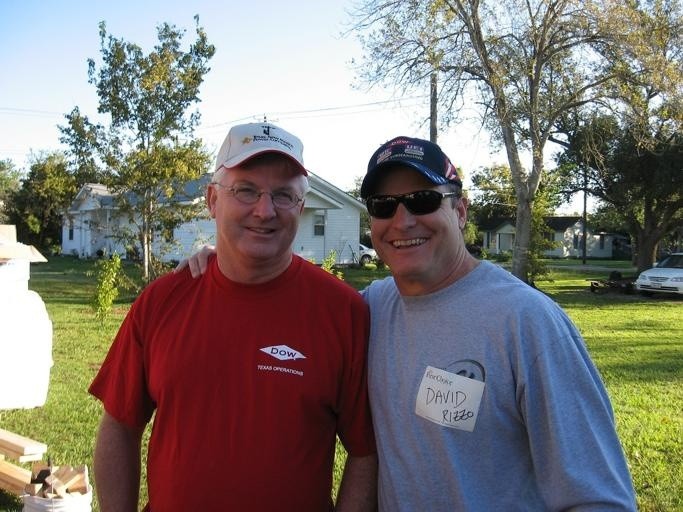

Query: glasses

(367, 191), (456, 219)
(214, 182), (303, 210)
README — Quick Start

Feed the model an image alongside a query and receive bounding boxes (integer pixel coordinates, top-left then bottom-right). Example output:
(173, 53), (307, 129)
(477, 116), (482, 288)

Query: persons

(169, 135), (638, 512)
(86, 122), (377, 512)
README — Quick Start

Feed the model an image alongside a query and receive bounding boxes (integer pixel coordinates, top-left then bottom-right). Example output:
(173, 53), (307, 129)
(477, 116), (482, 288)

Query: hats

(216, 122), (309, 174)
(360, 136), (462, 198)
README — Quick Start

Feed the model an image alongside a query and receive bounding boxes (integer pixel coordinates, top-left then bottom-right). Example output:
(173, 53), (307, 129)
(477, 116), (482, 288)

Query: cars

(359, 243), (379, 267)
(635, 253), (683, 298)
